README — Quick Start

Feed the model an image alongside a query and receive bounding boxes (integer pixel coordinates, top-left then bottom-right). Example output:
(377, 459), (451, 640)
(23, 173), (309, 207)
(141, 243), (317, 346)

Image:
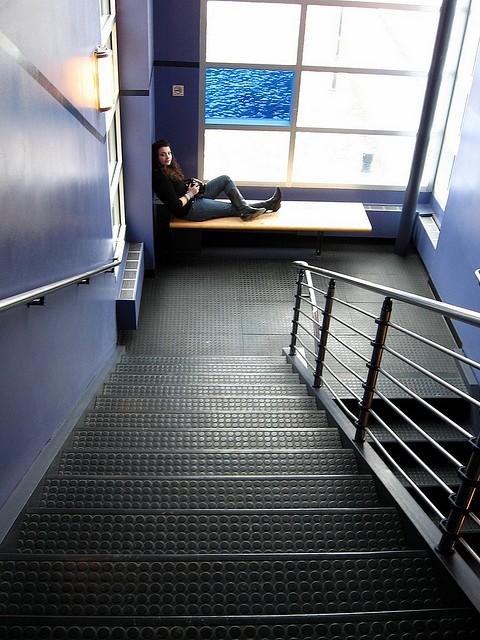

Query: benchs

(166, 199), (373, 253)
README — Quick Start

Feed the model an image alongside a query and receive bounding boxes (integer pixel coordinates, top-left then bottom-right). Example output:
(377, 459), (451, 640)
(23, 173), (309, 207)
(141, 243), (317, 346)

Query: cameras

(187, 177), (203, 191)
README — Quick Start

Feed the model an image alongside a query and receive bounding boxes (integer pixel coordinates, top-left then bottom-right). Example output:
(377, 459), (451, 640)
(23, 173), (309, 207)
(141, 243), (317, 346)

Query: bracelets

(184, 191), (194, 201)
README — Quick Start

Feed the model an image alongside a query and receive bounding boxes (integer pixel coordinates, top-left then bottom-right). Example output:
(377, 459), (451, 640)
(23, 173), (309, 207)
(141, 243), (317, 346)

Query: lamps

(96, 47), (113, 112)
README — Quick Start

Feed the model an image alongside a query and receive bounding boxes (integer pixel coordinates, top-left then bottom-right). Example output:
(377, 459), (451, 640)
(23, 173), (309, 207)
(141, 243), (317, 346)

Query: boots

(249, 187), (282, 212)
(227, 187), (266, 221)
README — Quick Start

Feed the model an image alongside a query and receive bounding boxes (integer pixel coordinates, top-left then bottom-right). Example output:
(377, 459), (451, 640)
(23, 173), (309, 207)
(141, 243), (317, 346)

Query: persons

(151, 139), (282, 222)
